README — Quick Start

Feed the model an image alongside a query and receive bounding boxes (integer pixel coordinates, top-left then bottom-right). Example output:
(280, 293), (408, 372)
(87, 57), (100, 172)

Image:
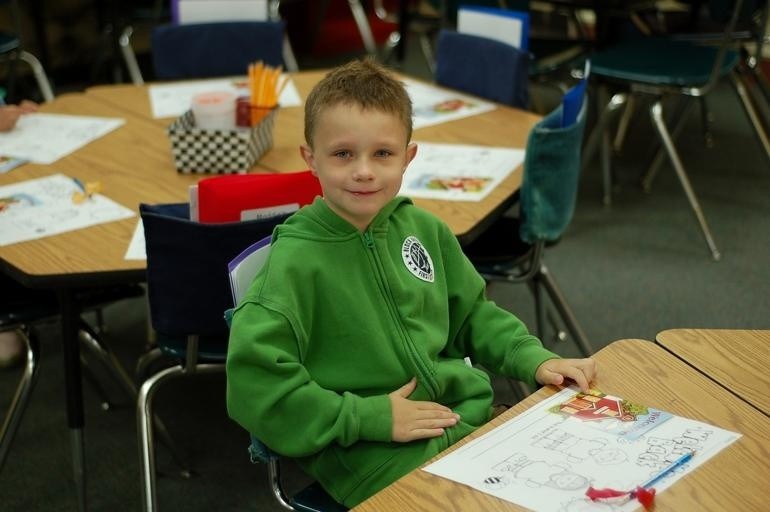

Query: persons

(226, 54), (596, 509)
(0, 98), (38, 130)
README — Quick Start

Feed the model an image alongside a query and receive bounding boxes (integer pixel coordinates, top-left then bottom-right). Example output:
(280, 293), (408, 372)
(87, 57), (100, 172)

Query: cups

(193, 92), (234, 131)
(237, 97), (278, 126)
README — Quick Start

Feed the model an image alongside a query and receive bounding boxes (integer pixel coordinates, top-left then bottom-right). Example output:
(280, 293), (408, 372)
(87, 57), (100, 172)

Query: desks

(656, 327), (770, 415)
(0, 93), (325, 512)
(347, 326), (769, 512)
(88, 59), (545, 246)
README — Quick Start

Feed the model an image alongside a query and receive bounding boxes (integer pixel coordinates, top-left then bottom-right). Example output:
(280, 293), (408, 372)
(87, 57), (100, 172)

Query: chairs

(223, 306), (347, 512)
(0, 263), (196, 482)
(145, 23), (291, 78)
(133, 211), (312, 512)
(462, 63), (596, 355)
(435, 32), (530, 107)
(577, 0), (770, 261)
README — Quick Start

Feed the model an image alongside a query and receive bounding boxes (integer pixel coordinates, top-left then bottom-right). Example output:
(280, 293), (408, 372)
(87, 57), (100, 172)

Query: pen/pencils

(617, 449), (697, 507)
(248, 61), (289, 127)
